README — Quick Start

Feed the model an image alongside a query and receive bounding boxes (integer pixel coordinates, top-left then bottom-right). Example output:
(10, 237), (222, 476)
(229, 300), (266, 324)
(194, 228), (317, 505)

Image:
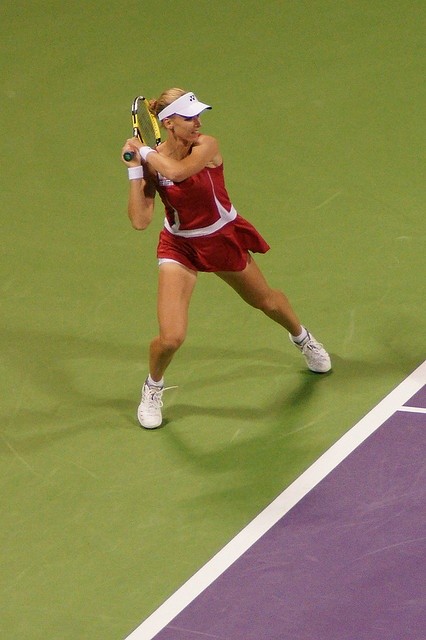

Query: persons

(119, 84), (332, 431)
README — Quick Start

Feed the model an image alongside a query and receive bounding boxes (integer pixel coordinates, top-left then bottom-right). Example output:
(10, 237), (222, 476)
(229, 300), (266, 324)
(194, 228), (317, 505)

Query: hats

(156, 91), (212, 121)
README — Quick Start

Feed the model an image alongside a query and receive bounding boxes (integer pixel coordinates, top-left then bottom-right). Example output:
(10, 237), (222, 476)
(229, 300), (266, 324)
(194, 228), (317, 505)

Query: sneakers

(288, 328), (331, 373)
(136, 377), (162, 428)
